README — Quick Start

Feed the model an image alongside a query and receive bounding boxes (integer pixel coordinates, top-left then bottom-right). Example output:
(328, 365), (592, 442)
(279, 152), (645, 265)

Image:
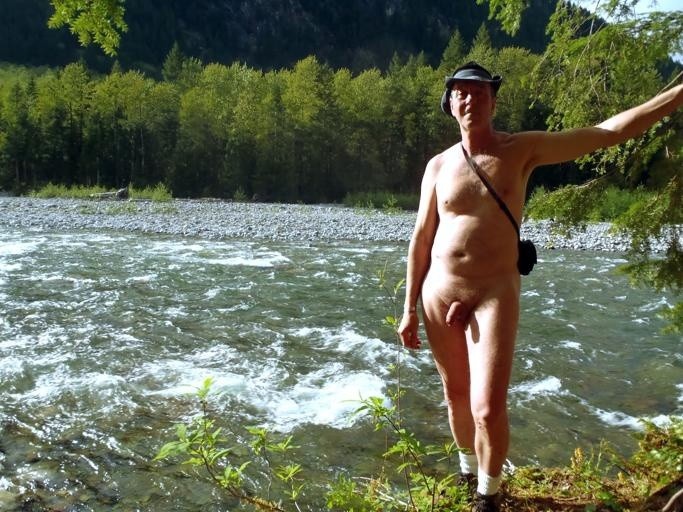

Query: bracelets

(403, 303), (416, 313)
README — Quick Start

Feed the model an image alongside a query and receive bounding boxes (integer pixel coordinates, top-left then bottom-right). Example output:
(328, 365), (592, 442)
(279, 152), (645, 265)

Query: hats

(440, 61), (502, 118)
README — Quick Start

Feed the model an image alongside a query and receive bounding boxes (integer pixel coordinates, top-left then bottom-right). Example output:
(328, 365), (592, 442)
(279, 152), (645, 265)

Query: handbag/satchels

(515, 238), (538, 276)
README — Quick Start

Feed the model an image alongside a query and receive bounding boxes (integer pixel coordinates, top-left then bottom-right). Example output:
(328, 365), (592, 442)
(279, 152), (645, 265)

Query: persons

(396, 63), (681, 511)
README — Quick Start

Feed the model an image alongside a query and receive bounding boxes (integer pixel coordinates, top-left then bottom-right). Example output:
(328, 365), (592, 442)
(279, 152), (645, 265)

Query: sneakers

(455, 472), (502, 512)
